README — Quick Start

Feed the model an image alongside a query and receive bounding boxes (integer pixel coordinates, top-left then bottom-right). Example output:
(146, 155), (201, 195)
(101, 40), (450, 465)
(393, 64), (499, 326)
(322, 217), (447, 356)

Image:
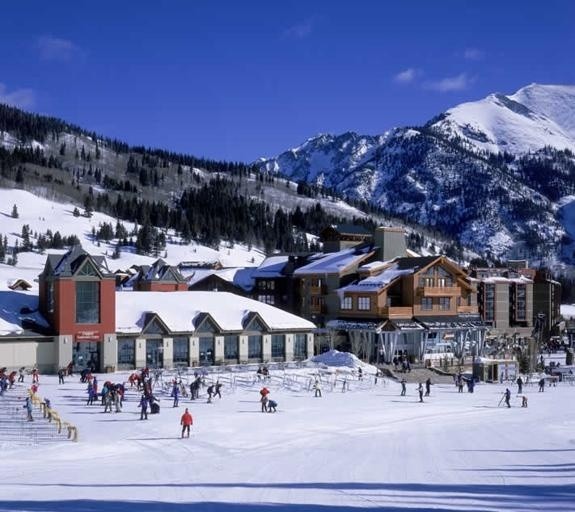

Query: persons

(57, 368), (65, 384)
(537, 378), (545, 392)
(137, 394), (151, 420)
(268, 399), (278, 413)
(393, 355), (411, 372)
(261, 395), (268, 412)
(516, 376), (524, 394)
(211, 380), (222, 398)
(457, 379), (465, 393)
(205, 384), (215, 403)
(315, 380), (322, 397)
(259, 387), (270, 400)
(262, 366), (269, 381)
(256, 366), (262, 382)
(180, 407), (193, 438)
(425, 377), (431, 396)
(170, 381), (179, 407)
(0, 366), (51, 422)
(400, 378), (408, 395)
(521, 396), (528, 407)
(188, 382), (196, 400)
(504, 387), (512, 407)
(416, 383), (424, 402)
(193, 377), (203, 398)
(79, 366), (161, 414)
(357, 366), (364, 382)
